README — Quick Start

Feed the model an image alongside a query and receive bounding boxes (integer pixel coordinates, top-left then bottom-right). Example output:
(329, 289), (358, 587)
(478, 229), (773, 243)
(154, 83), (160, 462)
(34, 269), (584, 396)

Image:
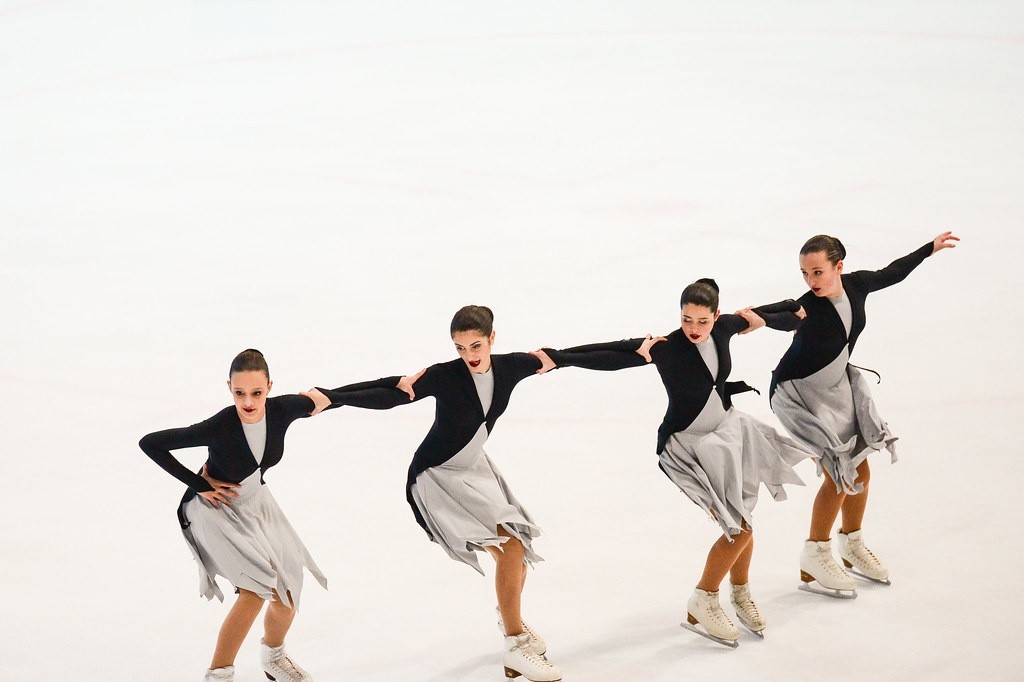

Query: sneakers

(838, 528), (890, 586)
(259, 642), (311, 682)
(495, 606), (546, 659)
(798, 539), (858, 599)
(680, 587), (739, 648)
(503, 634), (562, 682)
(730, 583), (766, 638)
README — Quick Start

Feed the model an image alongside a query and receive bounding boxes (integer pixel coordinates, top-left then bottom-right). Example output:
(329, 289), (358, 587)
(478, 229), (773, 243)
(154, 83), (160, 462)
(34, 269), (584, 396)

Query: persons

(300, 306), (668, 681)
(734, 231), (960, 599)
(139, 348), (426, 682)
(532, 278), (806, 648)
